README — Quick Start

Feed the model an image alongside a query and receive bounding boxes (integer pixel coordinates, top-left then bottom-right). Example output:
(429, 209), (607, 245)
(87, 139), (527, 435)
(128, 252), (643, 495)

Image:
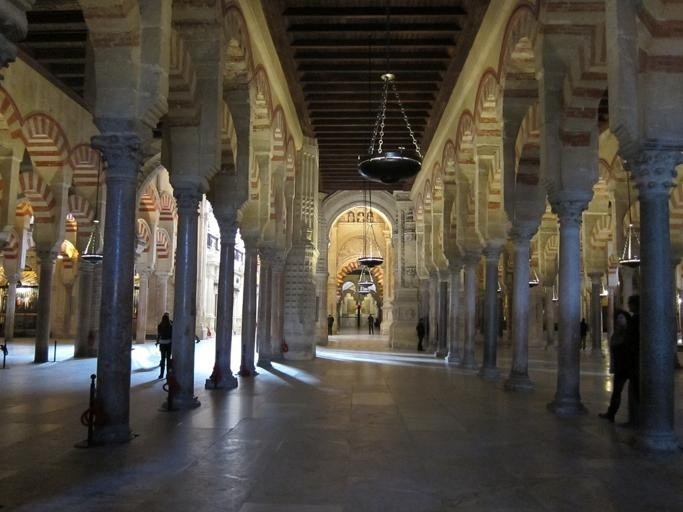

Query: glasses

(354, 1), (425, 184)
(80, 150), (108, 266)
(357, 181), (384, 268)
(527, 247), (540, 288)
(356, 262), (375, 295)
(617, 160), (640, 270)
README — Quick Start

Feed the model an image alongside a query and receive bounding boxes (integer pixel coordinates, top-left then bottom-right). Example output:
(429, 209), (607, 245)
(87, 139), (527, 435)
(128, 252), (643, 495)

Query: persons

(367, 313), (374, 334)
(373, 318), (379, 327)
(597, 295), (639, 429)
(158, 311), (173, 366)
(415, 316), (425, 352)
(327, 313), (334, 335)
(612, 307), (630, 344)
(579, 318), (587, 350)
(154, 314), (171, 379)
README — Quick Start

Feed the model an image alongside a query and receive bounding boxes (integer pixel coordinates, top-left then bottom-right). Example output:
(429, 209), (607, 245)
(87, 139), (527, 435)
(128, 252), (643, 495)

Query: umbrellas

(673, 363), (683, 369)
(598, 413), (615, 423)
